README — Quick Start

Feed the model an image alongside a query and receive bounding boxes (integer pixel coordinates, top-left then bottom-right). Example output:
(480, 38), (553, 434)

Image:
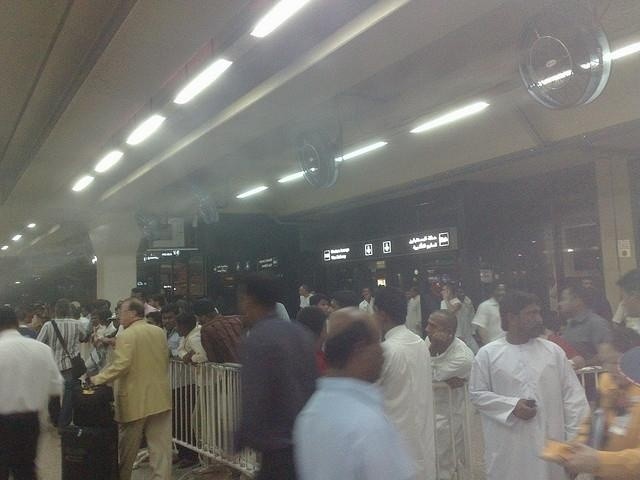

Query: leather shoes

(171, 454), (199, 468)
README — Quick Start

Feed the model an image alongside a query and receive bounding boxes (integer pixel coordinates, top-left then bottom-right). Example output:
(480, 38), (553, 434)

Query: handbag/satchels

(71, 384), (115, 427)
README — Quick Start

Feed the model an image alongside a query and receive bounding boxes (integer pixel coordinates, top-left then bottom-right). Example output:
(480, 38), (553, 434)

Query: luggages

(62, 421), (120, 480)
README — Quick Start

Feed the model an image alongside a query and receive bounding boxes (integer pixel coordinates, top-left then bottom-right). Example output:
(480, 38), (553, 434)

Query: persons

(359, 289), (438, 480)
(293, 306), (418, 479)
(541, 326), (640, 479)
(469, 290), (592, 479)
(84, 299), (174, 480)
(0, 257), (639, 480)
(414, 310), (478, 480)
(226, 272), (321, 479)
(1, 306), (63, 480)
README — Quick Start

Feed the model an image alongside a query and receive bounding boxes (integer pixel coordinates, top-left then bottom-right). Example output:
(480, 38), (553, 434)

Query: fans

(517, 1), (615, 113)
(135, 210), (169, 241)
(290, 119), (347, 193)
(179, 179), (231, 229)
